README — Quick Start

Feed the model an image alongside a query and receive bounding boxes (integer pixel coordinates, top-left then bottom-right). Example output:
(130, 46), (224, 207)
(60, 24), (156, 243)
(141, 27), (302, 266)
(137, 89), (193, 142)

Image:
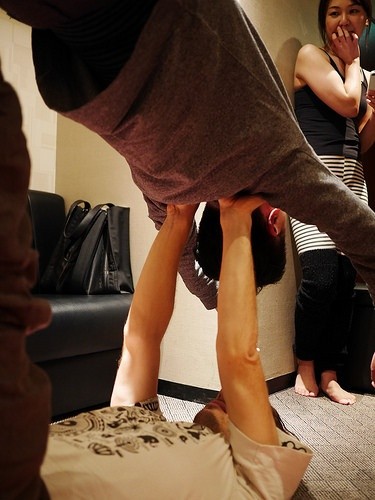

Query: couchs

(23, 189), (134, 423)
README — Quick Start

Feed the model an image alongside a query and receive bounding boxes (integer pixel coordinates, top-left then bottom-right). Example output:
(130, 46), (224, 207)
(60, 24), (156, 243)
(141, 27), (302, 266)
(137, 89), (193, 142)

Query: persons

(289, 0), (375, 406)
(0, 1), (375, 389)
(0, 64), (313, 500)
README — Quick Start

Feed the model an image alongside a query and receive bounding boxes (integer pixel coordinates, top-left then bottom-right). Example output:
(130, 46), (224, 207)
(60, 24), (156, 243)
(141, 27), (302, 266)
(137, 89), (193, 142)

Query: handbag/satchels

(53, 200), (134, 294)
